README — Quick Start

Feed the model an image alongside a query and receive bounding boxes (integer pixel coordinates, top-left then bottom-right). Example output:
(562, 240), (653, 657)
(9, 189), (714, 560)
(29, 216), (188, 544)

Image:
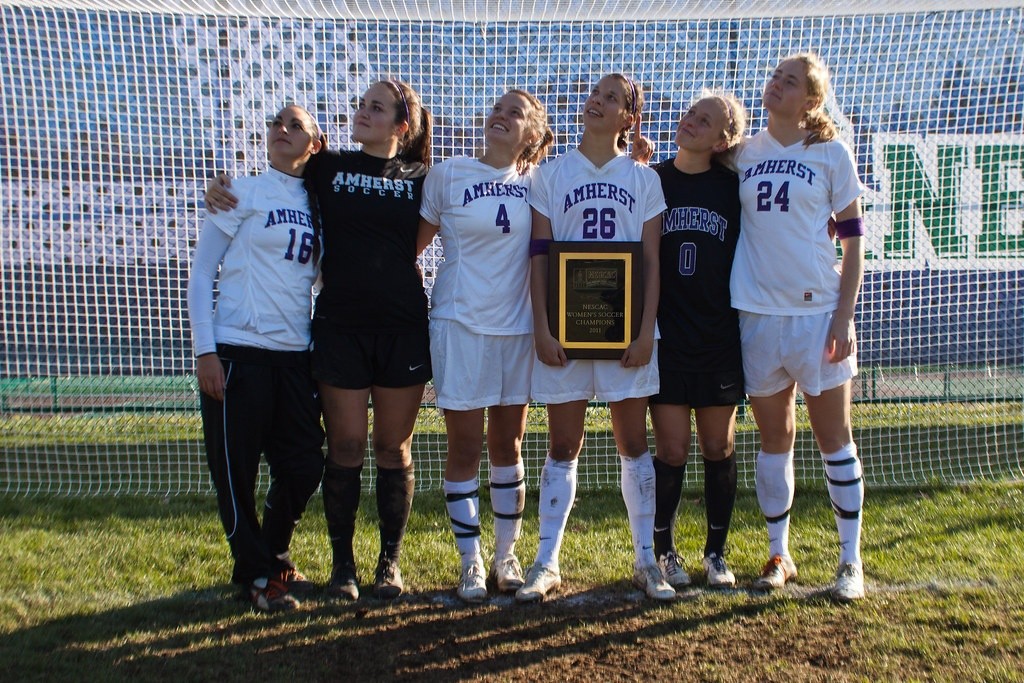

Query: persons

(515, 72), (677, 601)
(648, 55), (866, 602)
(187, 80), (433, 611)
(417, 90), (656, 603)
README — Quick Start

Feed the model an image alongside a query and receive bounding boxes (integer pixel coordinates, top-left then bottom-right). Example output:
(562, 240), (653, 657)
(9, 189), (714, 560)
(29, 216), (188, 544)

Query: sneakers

(490, 557), (526, 592)
(457, 558), (487, 603)
(656, 551), (692, 586)
(632, 563), (676, 600)
(514, 562), (561, 601)
(239, 578), (288, 614)
(832, 560), (865, 600)
(329, 562), (359, 601)
(372, 553), (403, 599)
(754, 553), (798, 590)
(276, 551), (314, 593)
(702, 548), (736, 588)
